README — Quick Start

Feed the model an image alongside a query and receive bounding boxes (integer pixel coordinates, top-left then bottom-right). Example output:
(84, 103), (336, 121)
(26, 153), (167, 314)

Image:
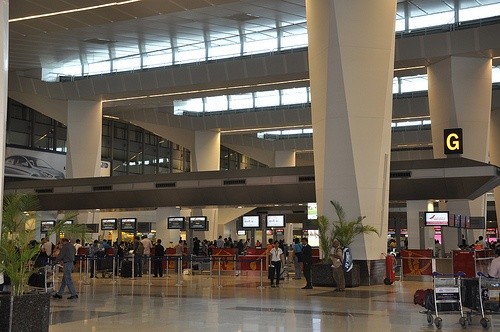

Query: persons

(269, 240), (286, 289)
(37, 231), (166, 280)
(174, 235), (295, 273)
(458, 235), (500, 251)
(330, 247), (346, 292)
(292, 238), (303, 279)
(52, 238), (80, 299)
(302, 237), (312, 289)
(434, 239), (441, 256)
(387, 237), (408, 257)
(488, 248), (500, 278)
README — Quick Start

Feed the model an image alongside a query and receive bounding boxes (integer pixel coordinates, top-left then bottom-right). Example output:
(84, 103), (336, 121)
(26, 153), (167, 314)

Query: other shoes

(271, 285), (275, 288)
(153, 276), (157, 277)
(67, 295), (78, 299)
(160, 275), (164, 277)
(53, 293), (63, 298)
(138, 275), (142, 277)
(302, 286), (312, 289)
(276, 285), (279, 288)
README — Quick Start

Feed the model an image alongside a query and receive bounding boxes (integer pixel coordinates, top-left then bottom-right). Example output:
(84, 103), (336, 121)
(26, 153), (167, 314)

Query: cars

(4, 155), (66, 180)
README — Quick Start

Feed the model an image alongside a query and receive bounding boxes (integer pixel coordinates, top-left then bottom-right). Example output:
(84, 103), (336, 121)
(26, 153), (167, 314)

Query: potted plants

(311, 200), (380, 286)
(0, 192), (94, 332)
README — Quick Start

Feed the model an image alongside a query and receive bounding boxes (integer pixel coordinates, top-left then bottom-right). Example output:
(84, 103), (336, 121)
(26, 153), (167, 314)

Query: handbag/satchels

(28, 272), (50, 288)
(137, 242), (144, 255)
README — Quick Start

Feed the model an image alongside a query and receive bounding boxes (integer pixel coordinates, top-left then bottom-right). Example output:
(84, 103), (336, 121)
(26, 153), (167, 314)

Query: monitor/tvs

(307, 202), (318, 220)
(266, 214), (285, 227)
(41, 221), (55, 232)
(242, 215), (261, 228)
(101, 219), (118, 230)
(425, 211), (450, 226)
(168, 217), (185, 229)
(189, 216), (208, 229)
(58, 220), (73, 225)
(121, 218), (136, 231)
